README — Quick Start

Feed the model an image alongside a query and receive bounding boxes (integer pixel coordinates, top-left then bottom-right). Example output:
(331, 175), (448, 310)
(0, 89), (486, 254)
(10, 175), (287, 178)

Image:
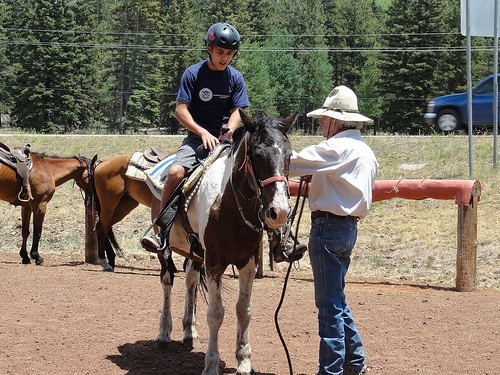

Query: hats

(307, 86), (374, 124)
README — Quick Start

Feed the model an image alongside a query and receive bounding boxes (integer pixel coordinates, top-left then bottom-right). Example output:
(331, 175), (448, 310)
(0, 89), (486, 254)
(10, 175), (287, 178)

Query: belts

(311, 210), (357, 221)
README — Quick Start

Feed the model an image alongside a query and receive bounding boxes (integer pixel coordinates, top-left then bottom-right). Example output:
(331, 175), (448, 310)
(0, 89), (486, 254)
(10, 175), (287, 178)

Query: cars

(423, 73), (500, 136)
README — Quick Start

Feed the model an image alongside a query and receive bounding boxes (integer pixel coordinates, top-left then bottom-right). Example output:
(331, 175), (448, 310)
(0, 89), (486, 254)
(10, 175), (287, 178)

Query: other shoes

(343, 364), (367, 373)
(275, 244), (307, 263)
(141, 236), (160, 253)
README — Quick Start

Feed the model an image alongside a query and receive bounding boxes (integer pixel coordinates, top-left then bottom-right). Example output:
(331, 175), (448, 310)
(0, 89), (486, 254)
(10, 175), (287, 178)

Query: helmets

(204, 23), (240, 50)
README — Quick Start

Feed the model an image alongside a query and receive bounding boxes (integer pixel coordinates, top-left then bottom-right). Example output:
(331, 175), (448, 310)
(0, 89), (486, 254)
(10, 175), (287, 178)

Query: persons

(289, 86), (378, 375)
(142, 22), (308, 264)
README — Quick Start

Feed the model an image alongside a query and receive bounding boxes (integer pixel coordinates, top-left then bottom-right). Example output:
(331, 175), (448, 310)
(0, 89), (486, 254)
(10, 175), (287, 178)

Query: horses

(0, 151), (99, 266)
(88, 155), (153, 271)
(148, 108), (300, 375)
